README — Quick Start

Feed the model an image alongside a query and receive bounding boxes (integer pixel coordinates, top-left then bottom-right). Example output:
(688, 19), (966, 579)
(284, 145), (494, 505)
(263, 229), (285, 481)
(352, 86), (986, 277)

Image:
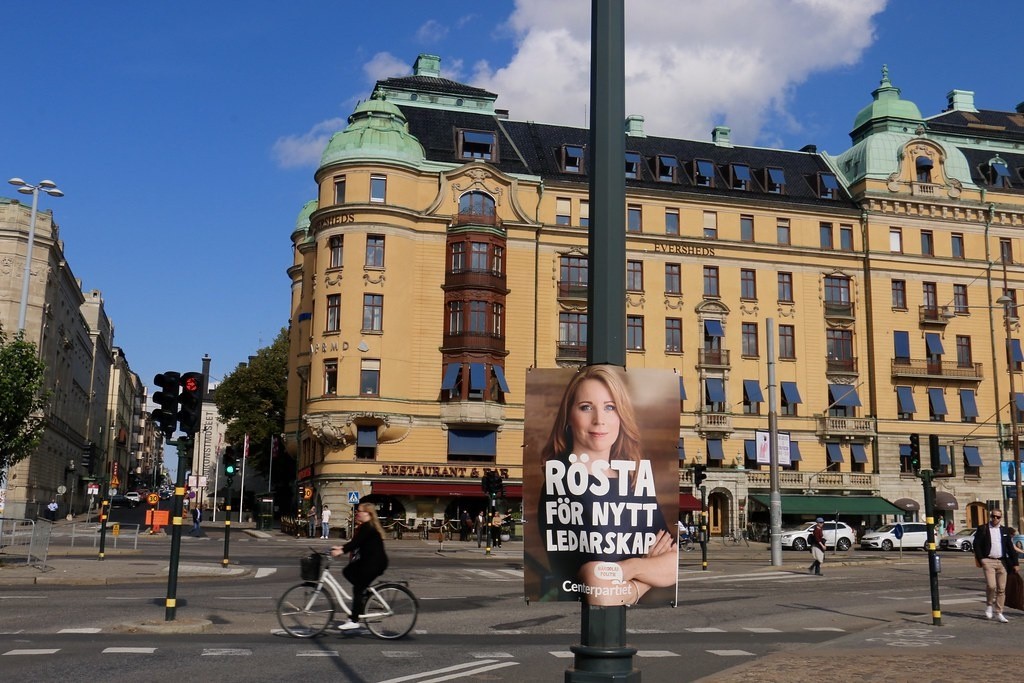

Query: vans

(860, 522), (941, 552)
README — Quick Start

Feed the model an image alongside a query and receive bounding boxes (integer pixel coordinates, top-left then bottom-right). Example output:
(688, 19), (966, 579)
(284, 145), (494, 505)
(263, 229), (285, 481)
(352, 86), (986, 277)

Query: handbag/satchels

(807, 534), (826, 553)
(304, 547), (327, 580)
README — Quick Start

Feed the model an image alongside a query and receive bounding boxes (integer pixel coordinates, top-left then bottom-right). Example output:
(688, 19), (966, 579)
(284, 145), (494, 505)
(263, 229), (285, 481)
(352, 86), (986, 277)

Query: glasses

(355, 509), (366, 514)
(990, 514), (1001, 520)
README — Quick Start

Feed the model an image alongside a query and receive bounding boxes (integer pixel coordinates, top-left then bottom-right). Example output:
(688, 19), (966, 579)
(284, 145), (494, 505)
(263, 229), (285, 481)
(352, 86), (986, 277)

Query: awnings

(370, 481), (523, 499)
(750, 493), (906, 516)
(676, 492), (709, 513)
(893, 497), (920, 511)
(933, 490), (958, 511)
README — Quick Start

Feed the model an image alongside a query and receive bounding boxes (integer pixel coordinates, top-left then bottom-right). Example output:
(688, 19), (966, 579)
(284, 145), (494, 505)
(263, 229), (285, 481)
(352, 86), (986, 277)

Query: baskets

(300, 559), (321, 580)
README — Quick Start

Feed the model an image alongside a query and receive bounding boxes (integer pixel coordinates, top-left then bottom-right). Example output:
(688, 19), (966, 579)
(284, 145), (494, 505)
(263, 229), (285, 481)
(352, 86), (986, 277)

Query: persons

(47, 499), (58, 524)
(678, 521), (688, 545)
(473, 508), (503, 547)
(973, 508), (1021, 623)
(307, 505), (318, 538)
(934, 515), (944, 537)
(331, 503), (389, 630)
(808, 517), (825, 576)
(945, 520), (955, 536)
(319, 505), (331, 540)
(534, 365), (677, 606)
(187, 503), (202, 538)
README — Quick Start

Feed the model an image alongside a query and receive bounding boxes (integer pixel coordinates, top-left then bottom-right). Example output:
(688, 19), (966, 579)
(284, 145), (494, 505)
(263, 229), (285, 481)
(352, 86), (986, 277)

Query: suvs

(769, 520), (856, 552)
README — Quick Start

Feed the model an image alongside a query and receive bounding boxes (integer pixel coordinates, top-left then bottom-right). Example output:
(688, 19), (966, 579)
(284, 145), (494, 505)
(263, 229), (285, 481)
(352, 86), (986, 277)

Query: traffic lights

(908, 433), (922, 469)
(175, 372), (204, 434)
(80, 442), (96, 475)
(224, 444), (237, 476)
(151, 371), (181, 433)
(234, 457), (241, 473)
(695, 463), (707, 485)
(492, 476), (503, 492)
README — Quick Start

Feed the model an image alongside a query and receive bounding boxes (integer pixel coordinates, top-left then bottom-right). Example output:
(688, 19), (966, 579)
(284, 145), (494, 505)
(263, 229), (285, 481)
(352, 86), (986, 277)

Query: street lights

(995, 296), (1024, 534)
(0, 177), (64, 557)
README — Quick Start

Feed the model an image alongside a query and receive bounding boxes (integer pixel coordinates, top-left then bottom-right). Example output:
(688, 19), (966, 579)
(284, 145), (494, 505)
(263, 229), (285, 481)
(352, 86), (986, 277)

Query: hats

(816, 518), (824, 523)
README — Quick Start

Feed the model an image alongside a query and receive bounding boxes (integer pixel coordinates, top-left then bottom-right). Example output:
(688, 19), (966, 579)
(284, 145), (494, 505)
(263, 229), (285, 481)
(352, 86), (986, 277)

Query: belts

(987, 557), (1002, 561)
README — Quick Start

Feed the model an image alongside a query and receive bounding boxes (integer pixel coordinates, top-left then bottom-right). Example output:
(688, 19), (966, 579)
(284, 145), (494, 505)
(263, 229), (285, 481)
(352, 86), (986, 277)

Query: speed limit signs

(147, 494), (158, 506)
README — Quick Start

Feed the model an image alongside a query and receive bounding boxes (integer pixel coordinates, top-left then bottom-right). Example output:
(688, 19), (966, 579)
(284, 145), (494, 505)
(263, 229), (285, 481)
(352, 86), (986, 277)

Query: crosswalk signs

(348, 489), (361, 505)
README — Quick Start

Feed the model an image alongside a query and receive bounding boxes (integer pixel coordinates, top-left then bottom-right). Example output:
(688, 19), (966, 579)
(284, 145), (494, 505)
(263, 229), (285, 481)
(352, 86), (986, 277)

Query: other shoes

(995, 614), (1008, 622)
(808, 567), (813, 573)
(816, 573), (823, 576)
(984, 606), (993, 619)
(338, 622), (360, 630)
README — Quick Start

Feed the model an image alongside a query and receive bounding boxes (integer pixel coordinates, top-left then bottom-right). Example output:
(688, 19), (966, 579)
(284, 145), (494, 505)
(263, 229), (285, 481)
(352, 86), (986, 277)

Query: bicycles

(276, 546), (419, 641)
(679, 536), (695, 552)
(723, 529), (751, 548)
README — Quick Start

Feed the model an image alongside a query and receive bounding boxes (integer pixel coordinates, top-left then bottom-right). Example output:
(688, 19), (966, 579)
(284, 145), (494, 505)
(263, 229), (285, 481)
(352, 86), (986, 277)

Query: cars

(124, 492), (143, 504)
(111, 494), (137, 508)
(939, 527), (977, 552)
(137, 484), (175, 501)
